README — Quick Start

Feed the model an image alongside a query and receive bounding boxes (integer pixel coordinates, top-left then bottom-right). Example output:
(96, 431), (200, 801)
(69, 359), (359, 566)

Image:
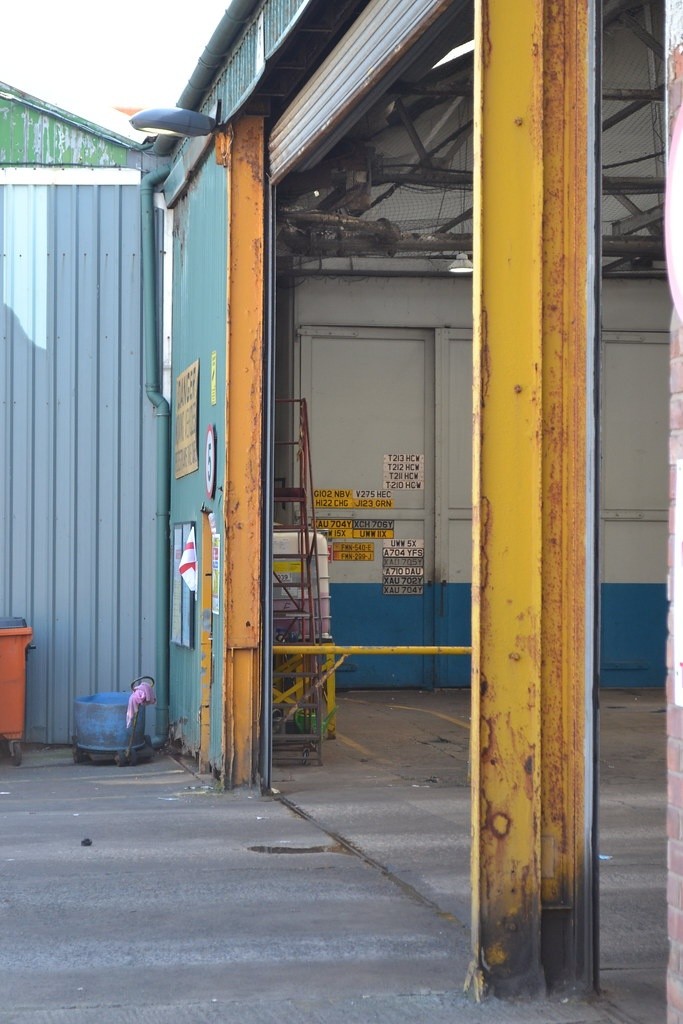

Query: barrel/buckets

(271, 532), (332, 639)
(272, 709), (285, 735)
(72, 676), (155, 762)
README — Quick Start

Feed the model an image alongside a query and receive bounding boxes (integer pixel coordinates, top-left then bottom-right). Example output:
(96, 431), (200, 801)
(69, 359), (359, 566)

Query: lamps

(129, 99), (228, 137)
(448, 251), (473, 273)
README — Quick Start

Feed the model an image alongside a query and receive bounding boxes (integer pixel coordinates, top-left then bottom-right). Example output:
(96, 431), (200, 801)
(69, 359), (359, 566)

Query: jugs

(295, 706), (338, 743)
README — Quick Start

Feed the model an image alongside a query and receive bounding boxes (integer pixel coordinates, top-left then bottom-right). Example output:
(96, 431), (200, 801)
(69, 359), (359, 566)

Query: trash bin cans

(0, 618), (33, 766)
